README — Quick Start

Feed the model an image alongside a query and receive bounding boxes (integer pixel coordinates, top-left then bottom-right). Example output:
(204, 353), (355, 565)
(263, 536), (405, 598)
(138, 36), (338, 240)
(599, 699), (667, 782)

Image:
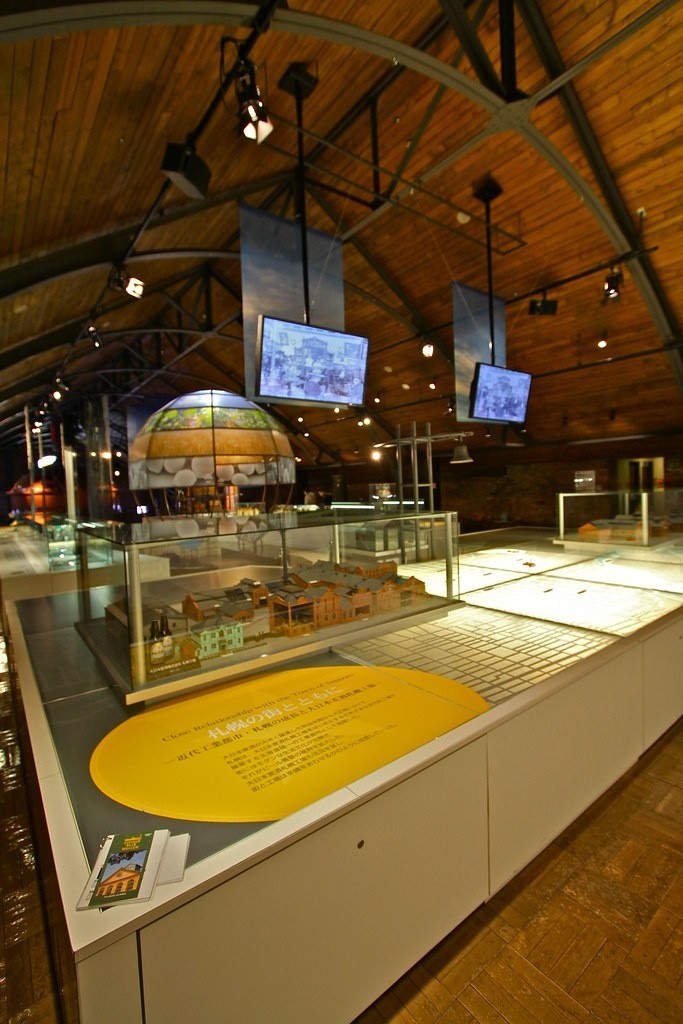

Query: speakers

(161, 142), (215, 198)
(529, 300), (558, 315)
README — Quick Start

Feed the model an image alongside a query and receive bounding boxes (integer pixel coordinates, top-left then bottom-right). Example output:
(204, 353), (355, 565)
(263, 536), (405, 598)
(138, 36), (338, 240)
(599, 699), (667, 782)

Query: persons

(304, 488), (316, 505)
(316, 489), (332, 508)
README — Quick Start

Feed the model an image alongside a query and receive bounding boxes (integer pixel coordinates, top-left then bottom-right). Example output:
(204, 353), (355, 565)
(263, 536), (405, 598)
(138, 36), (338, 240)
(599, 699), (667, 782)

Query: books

(76, 830), (191, 910)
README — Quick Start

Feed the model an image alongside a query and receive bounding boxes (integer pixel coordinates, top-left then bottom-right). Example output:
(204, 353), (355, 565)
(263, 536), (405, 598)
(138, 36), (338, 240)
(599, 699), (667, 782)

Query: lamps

(55, 371), (71, 392)
(449, 435), (474, 464)
(112, 259), (144, 300)
(527, 289), (557, 316)
(218, 37), (274, 145)
(161, 135), (211, 202)
(602, 265), (621, 306)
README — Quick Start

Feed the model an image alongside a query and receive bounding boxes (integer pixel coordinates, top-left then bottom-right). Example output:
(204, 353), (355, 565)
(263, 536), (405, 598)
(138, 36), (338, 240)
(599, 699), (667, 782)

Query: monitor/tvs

(254, 314), (369, 407)
(468, 362), (532, 425)
